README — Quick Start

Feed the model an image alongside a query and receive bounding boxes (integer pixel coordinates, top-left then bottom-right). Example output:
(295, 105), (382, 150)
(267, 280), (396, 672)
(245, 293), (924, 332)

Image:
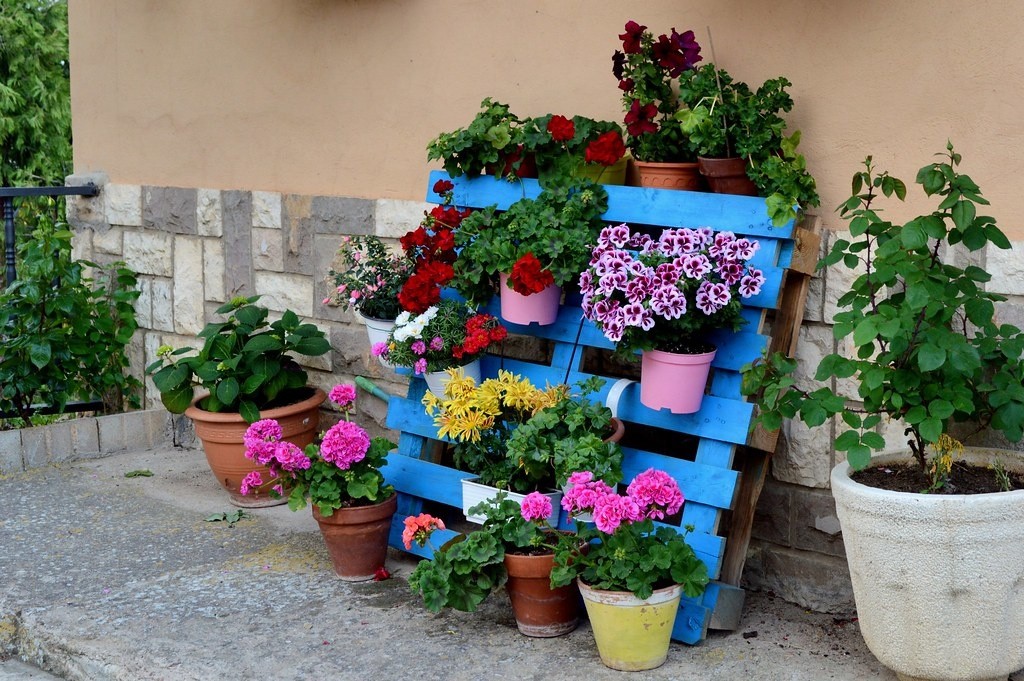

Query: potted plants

(737, 137), (1024, 681)
(425, 95), (538, 184)
(521, 113), (632, 185)
(143, 293), (336, 509)
(506, 375), (625, 488)
(673, 61), (820, 226)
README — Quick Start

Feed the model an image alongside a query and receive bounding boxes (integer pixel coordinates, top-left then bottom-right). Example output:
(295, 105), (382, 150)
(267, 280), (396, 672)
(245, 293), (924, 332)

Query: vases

(640, 342), (720, 414)
(503, 528), (589, 638)
(422, 356), (482, 401)
(577, 569), (685, 673)
(312, 490), (399, 582)
(461, 477), (563, 532)
(357, 304), (414, 368)
(633, 160), (707, 191)
(498, 270), (562, 324)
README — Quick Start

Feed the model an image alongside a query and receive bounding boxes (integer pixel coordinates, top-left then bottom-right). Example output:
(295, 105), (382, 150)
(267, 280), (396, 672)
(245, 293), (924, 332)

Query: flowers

(577, 220), (767, 363)
(371, 298), (508, 374)
(399, 480), (548, 614)
(239, 382), (399, 517)
(323, 232), (415, 317)
(611, 19), (703, 162)
(521, 467), (710, 599)
(421, 364), (584, 494)
(394, 113), (627, 316)
